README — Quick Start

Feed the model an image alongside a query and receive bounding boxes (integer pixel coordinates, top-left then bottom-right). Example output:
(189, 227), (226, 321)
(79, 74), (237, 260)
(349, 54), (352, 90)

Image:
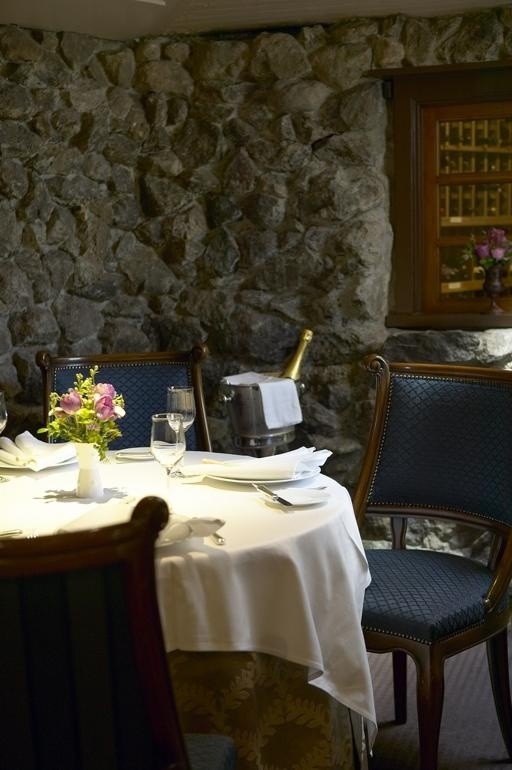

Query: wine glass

(165, 384), (198, 468)
(149, 413), (187, 498)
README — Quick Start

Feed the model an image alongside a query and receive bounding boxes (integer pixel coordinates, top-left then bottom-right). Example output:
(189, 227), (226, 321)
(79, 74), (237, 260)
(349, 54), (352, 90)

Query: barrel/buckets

(222, 375), (296, 449)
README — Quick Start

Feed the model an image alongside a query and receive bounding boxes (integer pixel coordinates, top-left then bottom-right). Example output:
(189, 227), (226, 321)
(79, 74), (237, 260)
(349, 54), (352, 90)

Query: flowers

(466, 219), (508, 270)
(35, 362), (126, 459)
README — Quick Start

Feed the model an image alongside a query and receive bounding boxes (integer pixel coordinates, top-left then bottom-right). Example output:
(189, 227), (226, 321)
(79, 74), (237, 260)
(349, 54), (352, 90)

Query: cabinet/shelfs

(357, 56), (510, 329)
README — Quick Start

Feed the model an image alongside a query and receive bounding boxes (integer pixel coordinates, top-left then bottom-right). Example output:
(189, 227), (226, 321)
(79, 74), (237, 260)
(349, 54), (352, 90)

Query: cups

(0, 389), (8, 435)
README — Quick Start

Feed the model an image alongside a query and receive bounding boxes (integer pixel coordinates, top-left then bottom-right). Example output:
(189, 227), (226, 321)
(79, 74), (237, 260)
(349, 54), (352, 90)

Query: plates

(201, 461), (321, 484)
(113, 445), (157, 461)
(257, 487), (333, 506)
(0, 456), (79, 469)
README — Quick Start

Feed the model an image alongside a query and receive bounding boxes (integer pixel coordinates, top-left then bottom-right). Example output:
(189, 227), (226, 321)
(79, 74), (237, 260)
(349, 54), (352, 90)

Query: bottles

(281, 328), (317, 380)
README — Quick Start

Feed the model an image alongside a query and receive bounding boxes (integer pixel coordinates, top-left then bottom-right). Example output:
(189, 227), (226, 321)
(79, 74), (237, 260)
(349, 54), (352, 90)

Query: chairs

(0, 495), (196, 768)
(34, 343), (221, 449)
(342, 348), (510, 767)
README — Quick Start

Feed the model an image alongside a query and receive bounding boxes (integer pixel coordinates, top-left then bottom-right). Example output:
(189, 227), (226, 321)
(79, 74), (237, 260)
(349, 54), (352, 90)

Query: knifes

(255, 484), (294, 508)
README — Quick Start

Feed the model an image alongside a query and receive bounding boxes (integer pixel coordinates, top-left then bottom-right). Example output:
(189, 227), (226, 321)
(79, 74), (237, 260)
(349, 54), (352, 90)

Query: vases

(486, 264), (502, 315)
(74, 440), (107, 503)
